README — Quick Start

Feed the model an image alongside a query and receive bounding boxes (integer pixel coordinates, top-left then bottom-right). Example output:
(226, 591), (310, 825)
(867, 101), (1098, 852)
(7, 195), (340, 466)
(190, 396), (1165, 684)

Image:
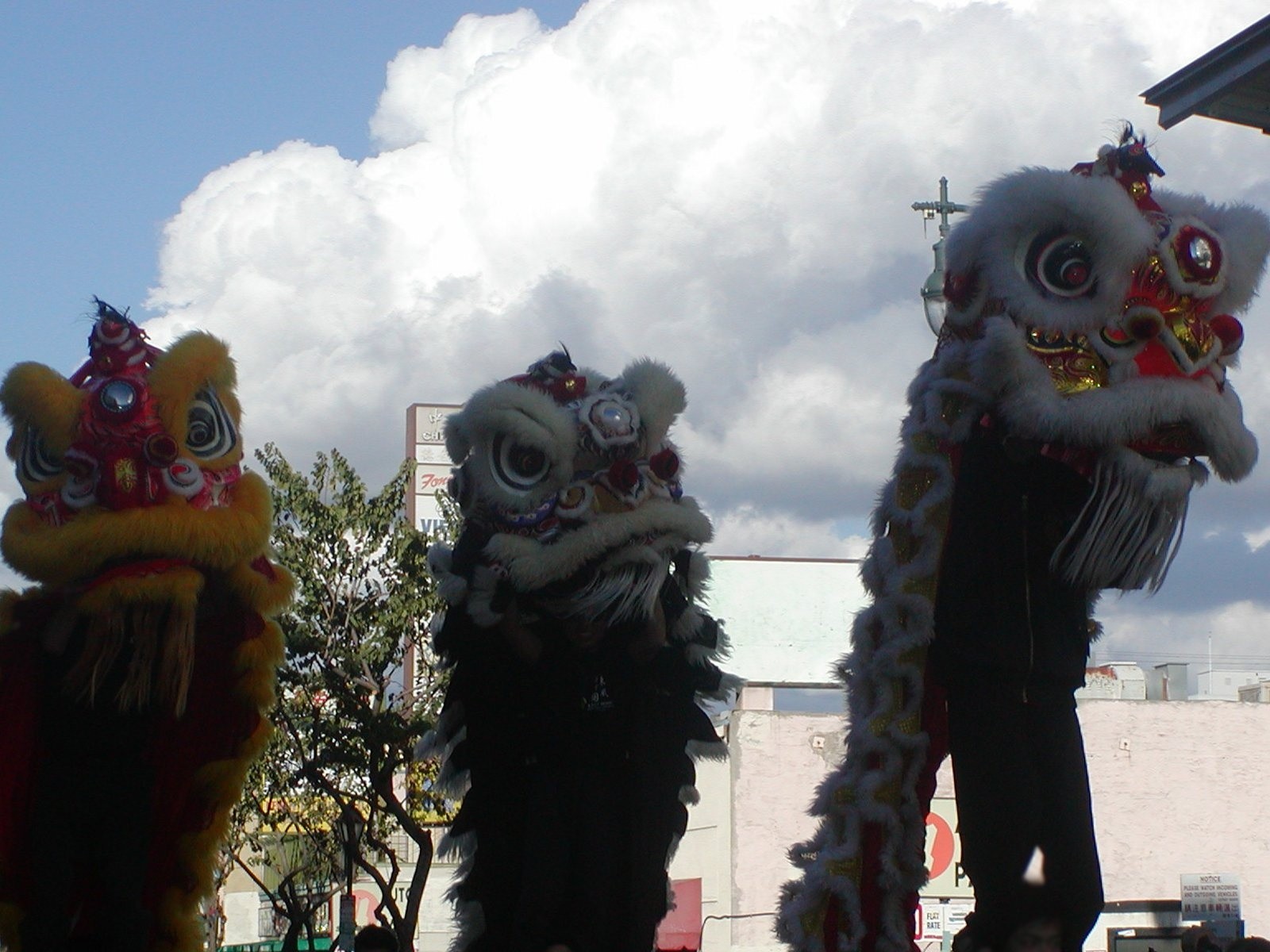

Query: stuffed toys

(0, 299), (296, 952)
(424, 350), (745, 952)
(777, 143), (1270, 952)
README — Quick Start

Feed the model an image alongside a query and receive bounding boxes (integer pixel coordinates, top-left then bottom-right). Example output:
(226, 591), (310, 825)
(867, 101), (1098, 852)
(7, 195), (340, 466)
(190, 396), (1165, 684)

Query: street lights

(334, 798), (366, 952)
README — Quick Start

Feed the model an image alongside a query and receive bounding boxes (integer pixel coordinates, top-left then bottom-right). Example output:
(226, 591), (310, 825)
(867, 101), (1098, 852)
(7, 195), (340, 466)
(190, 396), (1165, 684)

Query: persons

(354, 925), (401, 952)
(928, 463), (1104, 952)
